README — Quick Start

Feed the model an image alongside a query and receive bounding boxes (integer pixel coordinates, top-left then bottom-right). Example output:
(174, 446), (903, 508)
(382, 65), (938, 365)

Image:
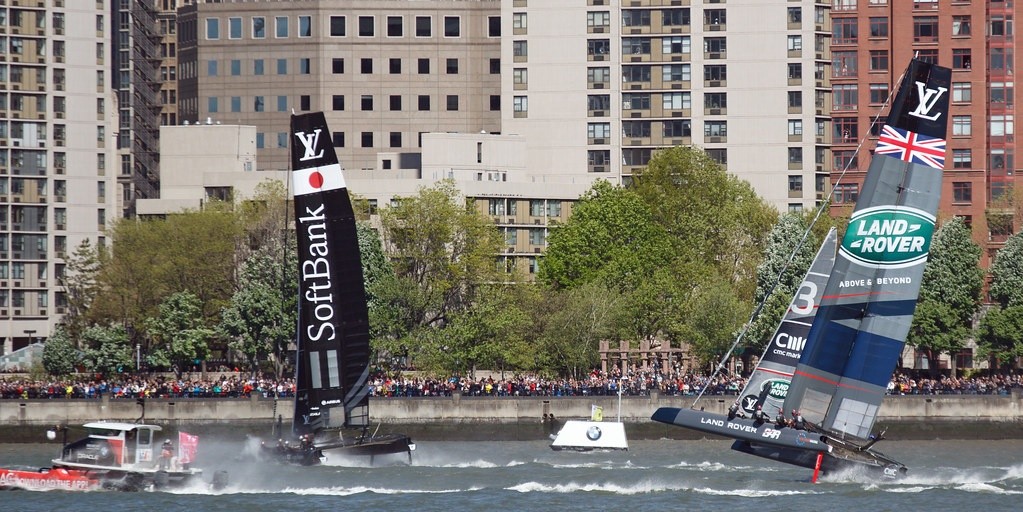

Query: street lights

(134, 343), (141, 371)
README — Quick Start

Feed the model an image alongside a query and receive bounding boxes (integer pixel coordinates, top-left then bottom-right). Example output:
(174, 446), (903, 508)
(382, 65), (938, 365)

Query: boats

(0, 396), (229, 492)
(549, 379), (629, 452)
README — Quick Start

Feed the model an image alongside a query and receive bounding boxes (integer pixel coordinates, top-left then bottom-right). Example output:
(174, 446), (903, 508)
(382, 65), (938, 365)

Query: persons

(776, 406), (790, 427)
(368, 362), (749, 393)
(789, 409), (812, 430)
(161, 439), (173, 470)
(729, 402), (746, 420)
(258, 434), (320, 464)
(1, 365), (296, 399)
(754, 404), (773, 423)
(543, 413), (560, 434)
(884, 369), (1023, 395)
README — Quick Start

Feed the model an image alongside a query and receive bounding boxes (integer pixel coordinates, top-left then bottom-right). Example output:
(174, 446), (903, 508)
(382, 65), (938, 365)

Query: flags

(178, 431), (200, 464)
(591, 404), (604, 420)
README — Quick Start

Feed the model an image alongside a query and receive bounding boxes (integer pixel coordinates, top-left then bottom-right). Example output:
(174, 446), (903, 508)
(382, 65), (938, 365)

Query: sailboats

(247, 108), (416, 468)
(652, 51), (952, 482)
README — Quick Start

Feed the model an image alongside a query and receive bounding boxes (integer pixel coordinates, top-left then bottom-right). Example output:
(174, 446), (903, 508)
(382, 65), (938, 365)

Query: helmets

(299, 435), (303, 440)
(779, 408), (783, 413)
(735, 401), (740, 405)
(261, 441), (264, 444)
(164, 439), (171, 444)
(757, 405), (762, 410)
(285, 441), (289, 444)
(304, 435), (308, 437)
(797, 410), (800, 415)
(279, 439), (283, 442)
(792, 409), (796, 415)
